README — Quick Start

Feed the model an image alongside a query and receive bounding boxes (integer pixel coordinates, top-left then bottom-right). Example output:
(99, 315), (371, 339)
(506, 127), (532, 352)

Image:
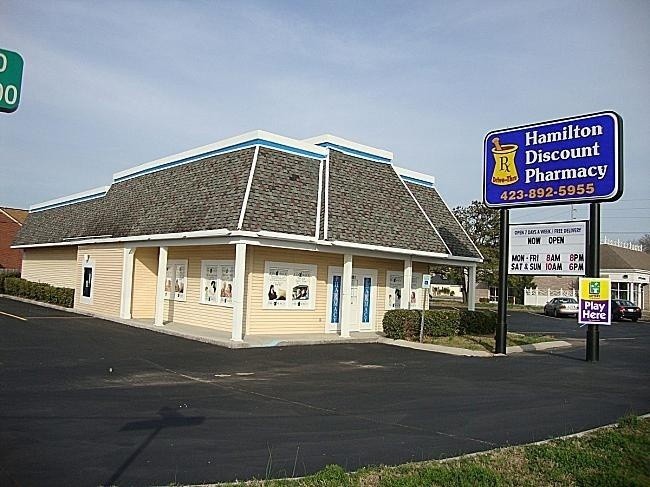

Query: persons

(204, 280), (232, 299)
(164, 279), (184, 293)
(268, 285), (277, 300)
(389, 289), (416, 308)
(295, 288), (307, 299)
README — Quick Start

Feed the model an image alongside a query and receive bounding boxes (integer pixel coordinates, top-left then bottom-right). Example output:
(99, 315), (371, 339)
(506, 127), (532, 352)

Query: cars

(543, 298), (579, 318)
(611, 299), (642, 322)
